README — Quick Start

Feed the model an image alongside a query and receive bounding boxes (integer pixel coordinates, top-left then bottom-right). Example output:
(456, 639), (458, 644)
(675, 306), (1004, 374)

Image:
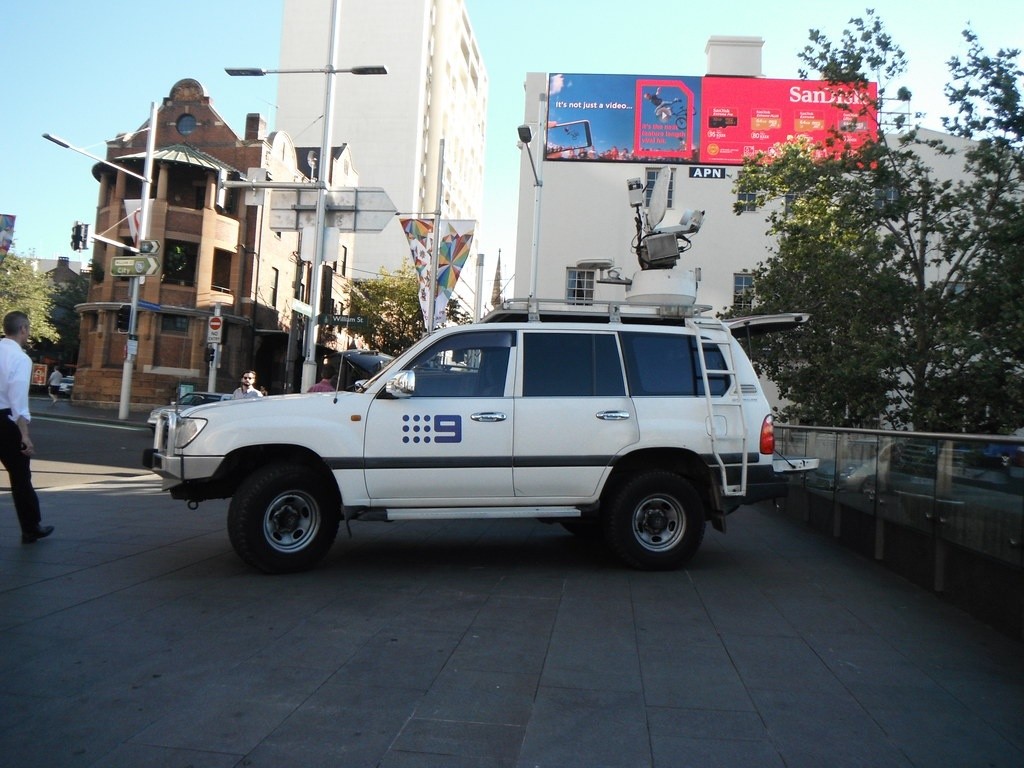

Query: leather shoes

(23, 525), (54, 543)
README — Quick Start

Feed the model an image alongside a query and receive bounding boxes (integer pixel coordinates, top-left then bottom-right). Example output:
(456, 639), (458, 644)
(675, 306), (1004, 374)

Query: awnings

(114, 142), (233, 172)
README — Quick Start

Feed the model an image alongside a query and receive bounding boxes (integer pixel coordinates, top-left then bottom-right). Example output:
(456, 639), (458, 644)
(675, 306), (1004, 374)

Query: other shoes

(53, 399), (57, 404)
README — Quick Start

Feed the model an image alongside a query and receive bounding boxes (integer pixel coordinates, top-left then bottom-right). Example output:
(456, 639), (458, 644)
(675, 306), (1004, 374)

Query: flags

(0, 214), (15, 264)
(401, 220), (475, 330)
(124, 201), (153, 247)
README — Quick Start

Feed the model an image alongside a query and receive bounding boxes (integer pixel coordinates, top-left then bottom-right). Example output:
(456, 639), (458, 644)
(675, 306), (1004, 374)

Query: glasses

(243, 376), (254, 380)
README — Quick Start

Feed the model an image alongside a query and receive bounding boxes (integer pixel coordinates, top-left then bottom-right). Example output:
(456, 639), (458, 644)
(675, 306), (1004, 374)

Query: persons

(260, 385), (268, 396)
(307, 365), (335, 392)
(233, 369), (264, 399)
(644, 87), (682, 112)
(1, 311), (54, 543)
(47, 366), (62, 403)
(567, 136), (694, 161)
